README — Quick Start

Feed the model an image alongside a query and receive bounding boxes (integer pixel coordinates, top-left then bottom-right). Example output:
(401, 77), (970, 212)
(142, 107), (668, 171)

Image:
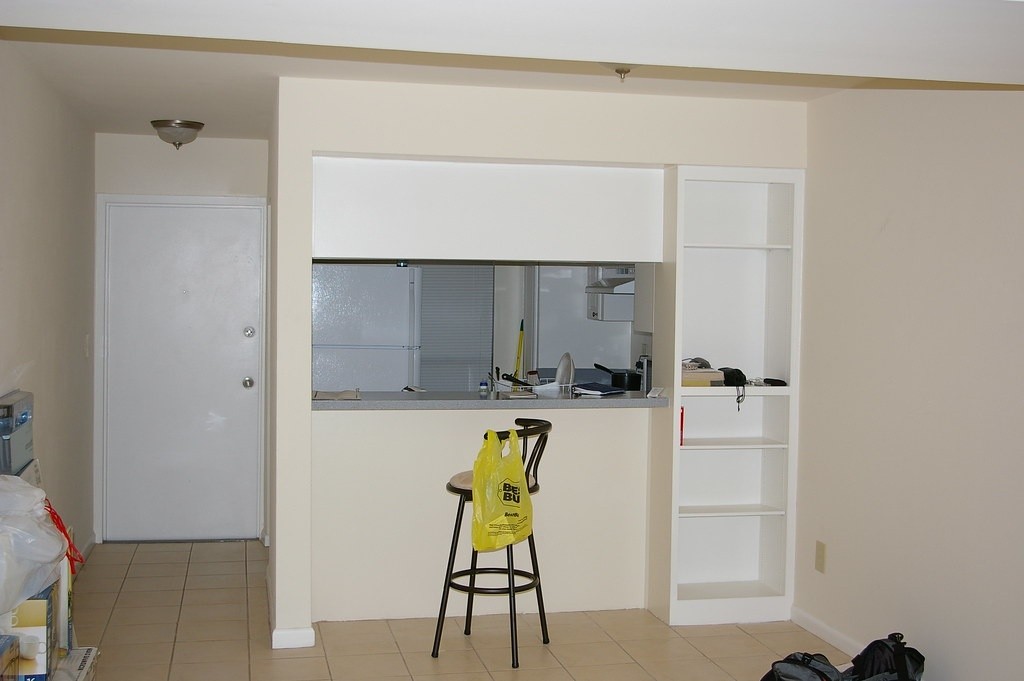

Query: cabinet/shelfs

(645, 162), (806, 626)
(633, 263), (654, 334)
(586, 293), (634, 322)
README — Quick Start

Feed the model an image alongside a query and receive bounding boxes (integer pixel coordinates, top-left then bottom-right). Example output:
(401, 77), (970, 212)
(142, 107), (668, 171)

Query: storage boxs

(52, 646), (98, 681)
(0, 580), (61, 681)
(59, 525), (73, 657)
(0, 635), (20, 681)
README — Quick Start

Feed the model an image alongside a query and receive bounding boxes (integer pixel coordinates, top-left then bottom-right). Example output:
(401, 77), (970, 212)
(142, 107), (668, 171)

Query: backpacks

(759, 633), (925, 681)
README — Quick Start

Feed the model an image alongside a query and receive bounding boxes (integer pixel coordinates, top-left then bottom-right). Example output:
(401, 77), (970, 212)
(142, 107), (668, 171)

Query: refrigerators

(311, 263), (421, 391)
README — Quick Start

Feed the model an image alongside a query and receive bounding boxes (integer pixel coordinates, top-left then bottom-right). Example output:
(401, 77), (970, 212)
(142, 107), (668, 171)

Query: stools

(431, 418), (553, 669)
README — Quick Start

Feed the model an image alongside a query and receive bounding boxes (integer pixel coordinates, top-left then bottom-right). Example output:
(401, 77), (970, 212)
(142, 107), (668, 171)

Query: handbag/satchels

(471, 429), (533, 553)
(718, 367), (746, 412)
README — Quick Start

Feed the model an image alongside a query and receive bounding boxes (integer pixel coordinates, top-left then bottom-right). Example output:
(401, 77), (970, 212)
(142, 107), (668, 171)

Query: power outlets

(815, 540), (827, 574)
(641, 344), (648, 355)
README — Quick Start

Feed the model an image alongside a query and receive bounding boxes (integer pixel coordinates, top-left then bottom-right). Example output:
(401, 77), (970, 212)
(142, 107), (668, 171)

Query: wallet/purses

(764, 379), (787, 386)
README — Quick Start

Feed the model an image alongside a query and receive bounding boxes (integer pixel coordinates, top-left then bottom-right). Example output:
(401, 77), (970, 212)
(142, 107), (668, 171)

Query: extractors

(585, 270), (635, 295)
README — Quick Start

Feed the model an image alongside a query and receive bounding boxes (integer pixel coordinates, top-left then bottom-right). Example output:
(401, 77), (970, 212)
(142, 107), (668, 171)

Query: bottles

(479, 381), (488, 389)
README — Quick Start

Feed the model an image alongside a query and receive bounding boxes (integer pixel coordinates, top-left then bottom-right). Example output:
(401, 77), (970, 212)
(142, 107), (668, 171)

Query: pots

(594, 363), (641, 390)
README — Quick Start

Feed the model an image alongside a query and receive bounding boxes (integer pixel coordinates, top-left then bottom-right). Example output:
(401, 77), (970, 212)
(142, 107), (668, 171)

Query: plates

(555, 352), (575, 384)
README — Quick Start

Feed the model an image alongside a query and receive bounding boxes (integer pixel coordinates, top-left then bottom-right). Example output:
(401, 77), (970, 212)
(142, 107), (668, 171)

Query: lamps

(150, 120), (205, 150)
